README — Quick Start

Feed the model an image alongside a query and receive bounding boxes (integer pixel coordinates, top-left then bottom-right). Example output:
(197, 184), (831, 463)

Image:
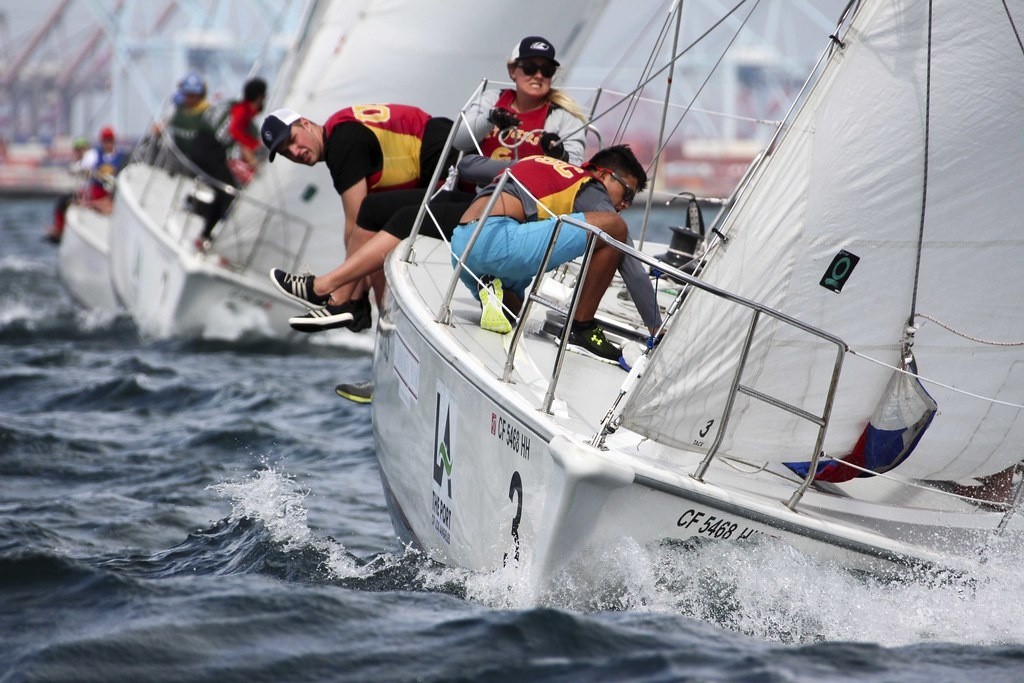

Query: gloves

(489, 106), (520, 132)
(540, 133), (565, 160)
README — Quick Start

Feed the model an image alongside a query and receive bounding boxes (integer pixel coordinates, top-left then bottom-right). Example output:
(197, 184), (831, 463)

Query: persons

(212, 77), (268, 149)
(173, 76), (236, 247)
(451, 144), (664, 364)
(83, 127), (123, 212)
(270, 36), (587, 335)
(261, 104), (432, 333)
(43, 138), (89, 238)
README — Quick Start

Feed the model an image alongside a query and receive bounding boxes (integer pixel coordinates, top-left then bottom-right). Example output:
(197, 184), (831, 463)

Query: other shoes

(40, 230), (60, 244)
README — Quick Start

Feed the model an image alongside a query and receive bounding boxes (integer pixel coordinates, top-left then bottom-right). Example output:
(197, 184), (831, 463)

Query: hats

(511, 36), (562, 66)
(173, 74), (208, 106)
(74, 137), (91, 149)
(260, 108), (302, 162)
(101, 127), (117, 139)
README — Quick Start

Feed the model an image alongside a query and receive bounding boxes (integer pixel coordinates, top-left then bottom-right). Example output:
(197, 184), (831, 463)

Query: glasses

(604, 168), (635, 205)
(514, 60), (558, 79)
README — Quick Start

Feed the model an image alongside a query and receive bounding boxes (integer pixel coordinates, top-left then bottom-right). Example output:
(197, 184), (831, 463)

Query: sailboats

(1, 0), (205, 202)
(110, 0), (605, 348)
(372, 1), (1024, 632)
(58, 0), (335, 325)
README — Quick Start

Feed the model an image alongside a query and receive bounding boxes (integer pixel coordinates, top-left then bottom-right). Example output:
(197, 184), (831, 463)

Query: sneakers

(335, 382), (374, 404)
(289, 302), (356, 333)
(270, 265), (331, 310)
(555, 316), (624, 365)
(477, 273), (512, 334)
